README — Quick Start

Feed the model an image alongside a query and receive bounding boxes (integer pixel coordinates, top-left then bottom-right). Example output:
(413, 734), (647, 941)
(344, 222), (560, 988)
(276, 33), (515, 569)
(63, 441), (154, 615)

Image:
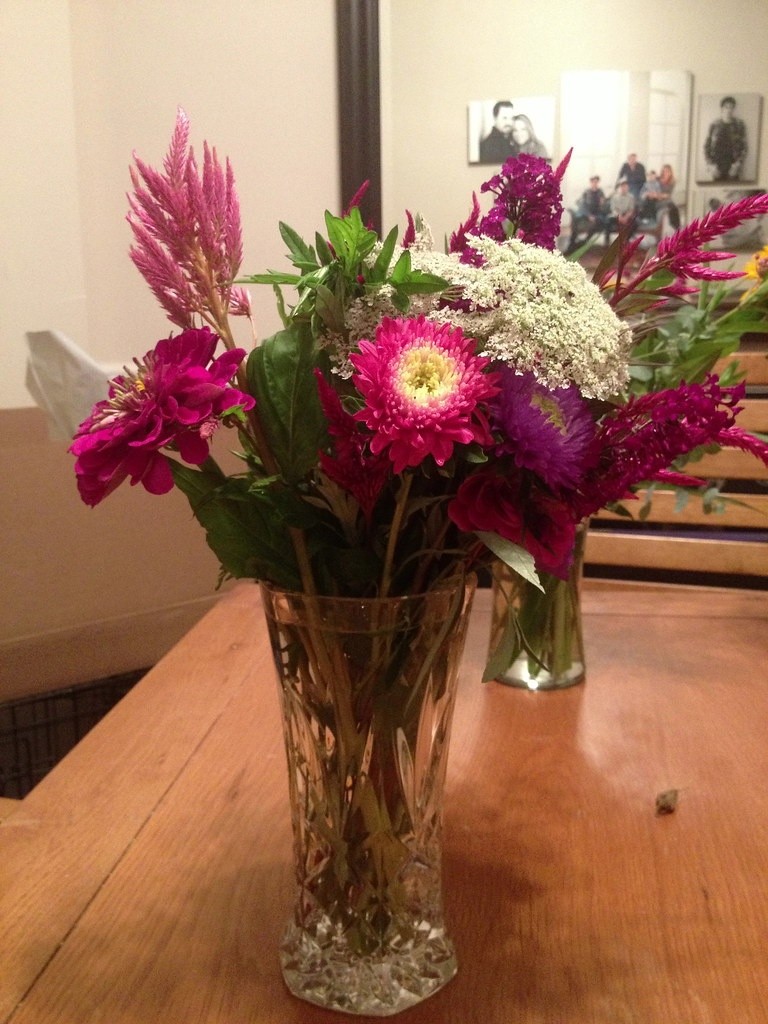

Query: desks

(0, 573), (768, 1024)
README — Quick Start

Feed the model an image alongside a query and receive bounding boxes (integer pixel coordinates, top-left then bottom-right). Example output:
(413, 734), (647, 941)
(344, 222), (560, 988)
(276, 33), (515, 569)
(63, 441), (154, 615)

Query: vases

(482, 511), (586, 692)
(255, 568), (479, 1017)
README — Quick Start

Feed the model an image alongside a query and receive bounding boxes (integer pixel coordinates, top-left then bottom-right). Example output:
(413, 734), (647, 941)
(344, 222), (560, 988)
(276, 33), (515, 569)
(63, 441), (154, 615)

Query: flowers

(76, 102), (768, 614)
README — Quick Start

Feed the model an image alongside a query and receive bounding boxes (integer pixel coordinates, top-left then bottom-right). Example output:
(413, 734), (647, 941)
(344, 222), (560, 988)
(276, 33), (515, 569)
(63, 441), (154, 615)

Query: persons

(576, 153), (680, 235)
(703, 97), (748, 180)
(480, 101), (547, 163)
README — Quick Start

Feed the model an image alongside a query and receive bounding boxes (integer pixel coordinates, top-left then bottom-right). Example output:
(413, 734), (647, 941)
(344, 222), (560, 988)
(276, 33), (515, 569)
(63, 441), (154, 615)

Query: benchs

(496, 351), (768, 596)
(564, 203), (671, 256)
